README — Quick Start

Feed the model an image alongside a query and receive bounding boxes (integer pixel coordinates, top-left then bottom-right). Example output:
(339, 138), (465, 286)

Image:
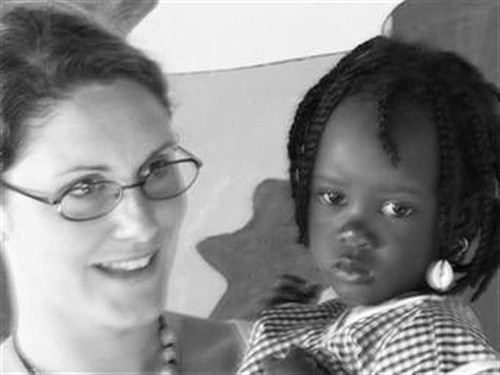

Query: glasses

(0, 144), (202, 223)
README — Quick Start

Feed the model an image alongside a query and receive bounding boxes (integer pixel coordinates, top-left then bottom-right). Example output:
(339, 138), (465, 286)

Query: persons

(1, 7), (255, 375)
(232, 35), (500, 375)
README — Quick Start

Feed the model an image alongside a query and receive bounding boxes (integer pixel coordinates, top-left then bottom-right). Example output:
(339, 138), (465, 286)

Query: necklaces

(11, 314), (180, 375)
